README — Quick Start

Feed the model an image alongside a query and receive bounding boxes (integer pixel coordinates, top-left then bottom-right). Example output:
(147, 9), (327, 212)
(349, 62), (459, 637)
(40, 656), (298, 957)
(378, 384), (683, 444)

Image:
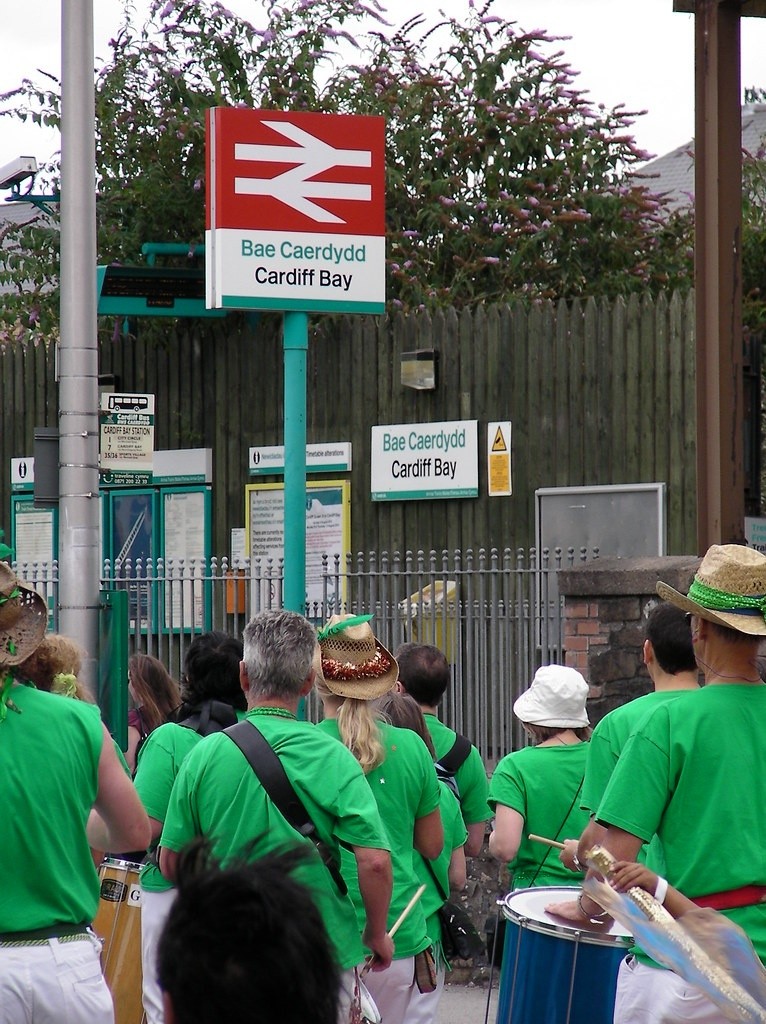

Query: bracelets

(572, 853), (583, 873)
(577, 893), (604, 917)
(654, 875), (668, 904)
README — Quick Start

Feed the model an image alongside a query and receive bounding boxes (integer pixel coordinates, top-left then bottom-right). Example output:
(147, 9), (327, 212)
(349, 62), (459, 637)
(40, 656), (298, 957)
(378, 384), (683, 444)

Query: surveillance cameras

(0, 156), (38, 190)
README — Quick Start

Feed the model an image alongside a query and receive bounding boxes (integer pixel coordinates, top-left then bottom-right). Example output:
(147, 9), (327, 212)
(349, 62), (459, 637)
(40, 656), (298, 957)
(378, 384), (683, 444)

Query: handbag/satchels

(485, 915), (507, 969)
(440, 901), (485, 959)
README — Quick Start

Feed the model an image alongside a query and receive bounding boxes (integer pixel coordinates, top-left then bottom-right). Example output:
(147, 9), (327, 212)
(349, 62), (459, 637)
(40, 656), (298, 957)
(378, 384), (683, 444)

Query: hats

(313, 614), (401, 702)
(656, 543), (766, 638)
(0, 560), (48, 666)
(511, 664), (590, 729)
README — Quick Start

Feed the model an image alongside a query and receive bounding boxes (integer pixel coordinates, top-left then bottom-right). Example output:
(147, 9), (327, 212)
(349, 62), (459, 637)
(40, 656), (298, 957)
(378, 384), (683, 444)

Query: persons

(561, 543), (765, 1024)
(129, 631), (247, 1024)
(154, 863), (343, 1024)
(24, 631), (184, 782)
(311, 615), (489, 1023)
(585, 602), (701, 884)
(0, 563), (149, 1023)
(158, 611), (393, 1024)
(608, 860), (700, 920)
(486, 663), (647, 904)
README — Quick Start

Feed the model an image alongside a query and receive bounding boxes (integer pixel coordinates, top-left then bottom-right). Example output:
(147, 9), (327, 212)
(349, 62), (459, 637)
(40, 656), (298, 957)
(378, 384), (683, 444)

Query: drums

(90, 856), (147, 1024)
(495, 885), (634, 1024)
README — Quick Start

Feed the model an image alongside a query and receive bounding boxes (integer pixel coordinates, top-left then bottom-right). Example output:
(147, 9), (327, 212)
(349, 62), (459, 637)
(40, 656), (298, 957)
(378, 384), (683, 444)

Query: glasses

(685, 611), (695, 627)
